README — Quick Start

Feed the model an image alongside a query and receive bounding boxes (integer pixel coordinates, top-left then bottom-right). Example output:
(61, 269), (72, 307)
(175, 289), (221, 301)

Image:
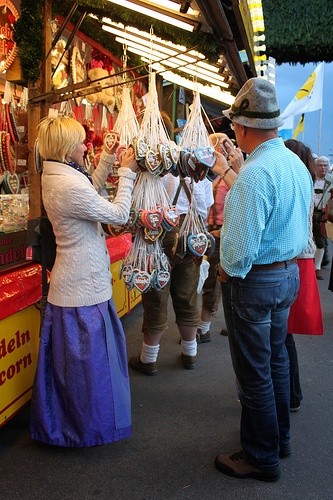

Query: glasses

(230, 122), (236, 131)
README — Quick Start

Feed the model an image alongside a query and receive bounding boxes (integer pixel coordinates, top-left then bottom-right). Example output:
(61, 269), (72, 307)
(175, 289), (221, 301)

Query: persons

(31, 116), (138, 448)
(211, 77), (314, 482)
(284, 138), (333, 293)
(279, 137), (322, 412)
(129, 173), (201, 376)
(178, 133), (233, 345)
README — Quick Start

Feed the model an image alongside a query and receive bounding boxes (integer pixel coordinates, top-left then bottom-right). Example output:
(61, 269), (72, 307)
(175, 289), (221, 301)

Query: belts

(252, 257), (299, 271)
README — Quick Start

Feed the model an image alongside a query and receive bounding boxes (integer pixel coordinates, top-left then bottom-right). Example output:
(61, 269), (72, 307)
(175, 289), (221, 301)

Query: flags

(280, 61), (324, 130)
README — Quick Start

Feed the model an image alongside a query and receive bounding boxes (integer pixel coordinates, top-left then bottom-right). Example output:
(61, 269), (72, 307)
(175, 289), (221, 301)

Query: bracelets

(221, 167), (232, 180)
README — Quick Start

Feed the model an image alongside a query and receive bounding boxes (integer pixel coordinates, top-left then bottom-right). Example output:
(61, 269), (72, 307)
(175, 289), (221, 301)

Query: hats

(222, 77), (284, 129)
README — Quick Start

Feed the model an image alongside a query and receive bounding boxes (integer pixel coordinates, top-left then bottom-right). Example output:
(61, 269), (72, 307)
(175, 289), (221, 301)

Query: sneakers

(220, 328), (228, 336)
(278, 444), (293, 456)
(129, 355), (157, 376)
(180, 352), (197, 370)
(179, 329), (211, 344)
(215, 449), (281, 482)
(286, 394), (301, 411)
(315, 269), (324, 280)
